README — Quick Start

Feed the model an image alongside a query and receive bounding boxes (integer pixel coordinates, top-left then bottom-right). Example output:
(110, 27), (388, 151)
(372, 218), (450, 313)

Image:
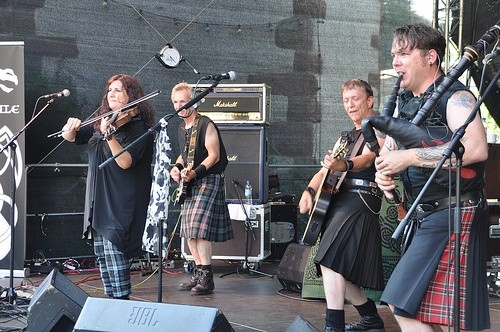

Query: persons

(170, 80), (234, 295)
(62, 74), (156, 301)
(298, 78), (385, 332)
(374, 23), (491, 332)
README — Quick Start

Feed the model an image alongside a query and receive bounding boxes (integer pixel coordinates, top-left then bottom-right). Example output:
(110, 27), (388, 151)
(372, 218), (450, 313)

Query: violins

(92, 107), (140, 142)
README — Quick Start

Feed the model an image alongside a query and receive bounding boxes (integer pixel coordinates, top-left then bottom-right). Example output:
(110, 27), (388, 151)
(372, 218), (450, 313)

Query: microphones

(232, 179), (240, 187)
(40, 89), (70, 99)
(201, 71), (236, 80)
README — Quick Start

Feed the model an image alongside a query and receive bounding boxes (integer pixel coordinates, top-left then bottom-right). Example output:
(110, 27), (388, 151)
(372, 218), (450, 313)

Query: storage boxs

(180, 204), (272, 275)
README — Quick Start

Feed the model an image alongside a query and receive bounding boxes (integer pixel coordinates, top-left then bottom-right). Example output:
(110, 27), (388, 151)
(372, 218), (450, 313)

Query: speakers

(276, 243), (314, 293)
(72, 297), (236, 332)
(216, 126), (269, 205)
(285, 314), (321, 332)
(26, 268), (90, 332)
(262, 204), (298, 262)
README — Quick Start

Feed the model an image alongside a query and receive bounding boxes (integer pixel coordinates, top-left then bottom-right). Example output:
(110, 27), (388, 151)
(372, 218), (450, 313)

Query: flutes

(361, 17), (500, 222)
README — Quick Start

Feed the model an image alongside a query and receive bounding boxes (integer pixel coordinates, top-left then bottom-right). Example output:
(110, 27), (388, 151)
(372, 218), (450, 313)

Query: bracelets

(304, 187), (316, 196)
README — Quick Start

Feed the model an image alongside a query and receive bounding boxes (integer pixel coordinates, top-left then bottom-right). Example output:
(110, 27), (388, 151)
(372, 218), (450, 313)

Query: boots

(179, 265), (214, 295)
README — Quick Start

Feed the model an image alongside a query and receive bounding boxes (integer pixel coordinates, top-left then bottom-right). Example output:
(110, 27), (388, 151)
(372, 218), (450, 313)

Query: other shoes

(344, 313), (385, 331)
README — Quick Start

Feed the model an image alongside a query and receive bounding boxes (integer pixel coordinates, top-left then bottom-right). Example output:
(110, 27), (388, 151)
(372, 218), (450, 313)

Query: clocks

(156, 43), (186, 68)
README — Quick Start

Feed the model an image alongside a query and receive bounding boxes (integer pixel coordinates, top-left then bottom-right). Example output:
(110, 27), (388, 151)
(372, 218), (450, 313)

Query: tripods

(218, 185), (274, 279)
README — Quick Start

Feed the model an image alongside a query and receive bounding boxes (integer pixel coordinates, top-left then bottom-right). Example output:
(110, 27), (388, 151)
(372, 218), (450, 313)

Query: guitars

(304, 137), (349, 247)
(171, 161), (194, 208)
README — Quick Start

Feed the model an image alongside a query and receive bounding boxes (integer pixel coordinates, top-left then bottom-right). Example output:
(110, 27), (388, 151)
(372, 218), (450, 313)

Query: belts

(412, 186), (482, 212)
(340, 177), (384, 195)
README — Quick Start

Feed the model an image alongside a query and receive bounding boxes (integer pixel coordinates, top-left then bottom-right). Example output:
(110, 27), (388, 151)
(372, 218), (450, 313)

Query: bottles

(244, 180), (252, 205)
